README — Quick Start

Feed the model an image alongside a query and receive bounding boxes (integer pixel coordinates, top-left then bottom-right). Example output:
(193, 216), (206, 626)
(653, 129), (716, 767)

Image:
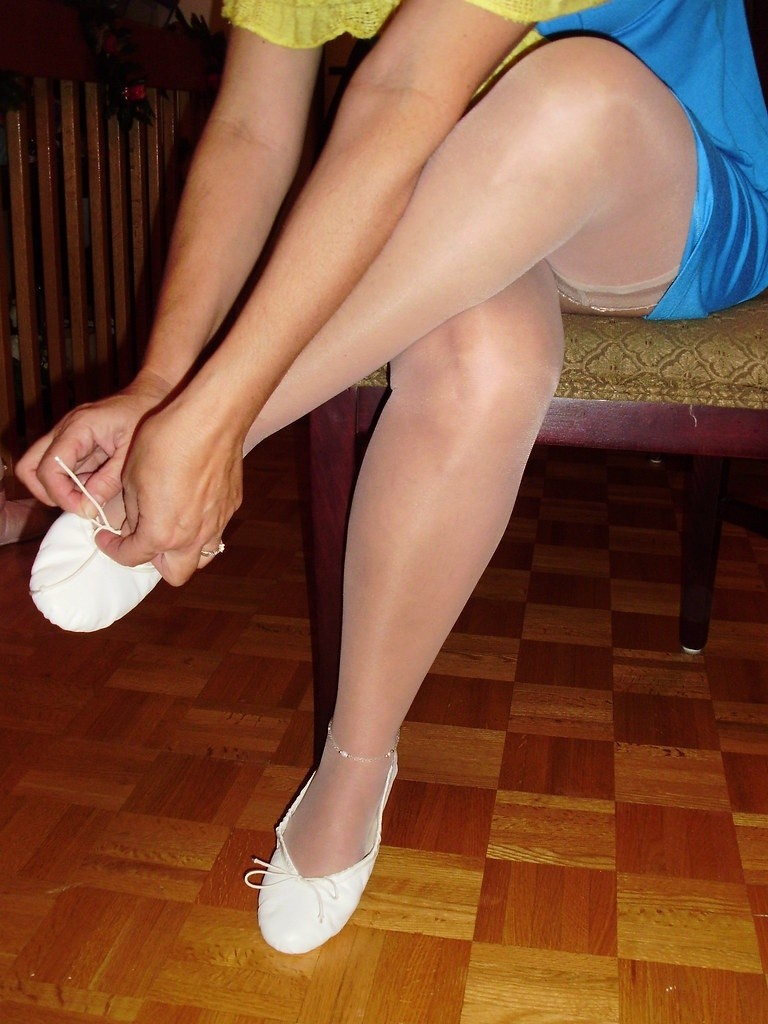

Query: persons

(17, 0), (768, 953)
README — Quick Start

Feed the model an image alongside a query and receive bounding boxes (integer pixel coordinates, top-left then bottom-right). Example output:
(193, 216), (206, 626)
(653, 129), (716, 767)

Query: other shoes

(28, 456), (165, 633)
(243, 745), (398, 954)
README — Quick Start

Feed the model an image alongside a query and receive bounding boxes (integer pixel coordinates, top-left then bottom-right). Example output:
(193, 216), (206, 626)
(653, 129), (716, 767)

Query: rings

(198, 537), (226, 559)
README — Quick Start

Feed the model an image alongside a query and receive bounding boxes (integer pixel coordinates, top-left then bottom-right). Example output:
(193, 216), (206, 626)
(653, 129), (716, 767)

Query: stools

(310, 298), (768, 778)
